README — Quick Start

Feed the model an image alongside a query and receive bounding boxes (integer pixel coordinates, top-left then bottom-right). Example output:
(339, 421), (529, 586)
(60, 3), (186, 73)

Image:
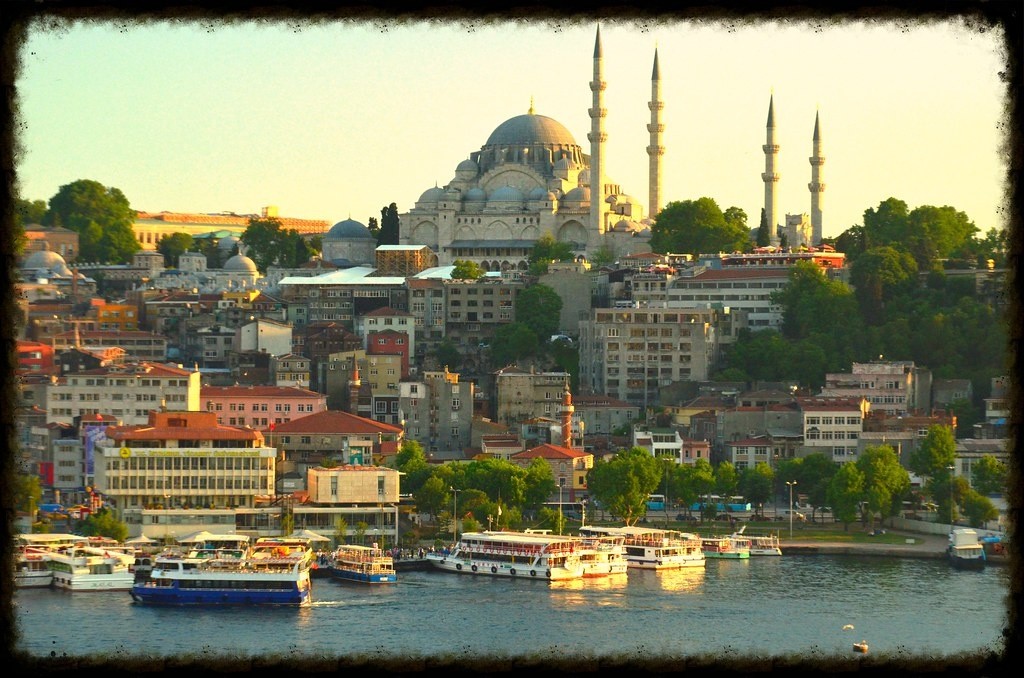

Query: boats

(11, 537), (314, 607)
(326, 545), (398, 585)
(946, 529), (986, 571)
(426, 524), (782, 581)
(983, 533), (1012, 565)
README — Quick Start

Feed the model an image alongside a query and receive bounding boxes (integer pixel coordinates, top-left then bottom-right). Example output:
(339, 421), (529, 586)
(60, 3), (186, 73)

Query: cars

(750, 514), (771, 523)
(676, 513), (698, 521)
(715, 514), (740, 522)
(36, 503), (89, 522)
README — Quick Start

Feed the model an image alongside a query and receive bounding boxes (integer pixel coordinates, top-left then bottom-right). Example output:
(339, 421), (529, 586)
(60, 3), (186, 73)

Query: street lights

(452, 488), (461, 550)
(786, 481), (798, 539)
(390, 503), (398, 547)
(555, 484), (562, 536)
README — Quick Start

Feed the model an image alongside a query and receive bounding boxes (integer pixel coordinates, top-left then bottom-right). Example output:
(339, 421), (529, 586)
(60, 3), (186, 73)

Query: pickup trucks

(777, 510), (804, 521)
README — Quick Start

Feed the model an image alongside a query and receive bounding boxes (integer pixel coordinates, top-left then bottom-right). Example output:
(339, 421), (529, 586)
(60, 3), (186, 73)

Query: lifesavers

(472, 565), (477, 571)
(546, 571), (551, 577)
(510, 569), (516, 574)
(491, 566), (497, 573)
(530, 570), (535, 576)
(456, 564), (461, 570)
(440, 560), (444, 564)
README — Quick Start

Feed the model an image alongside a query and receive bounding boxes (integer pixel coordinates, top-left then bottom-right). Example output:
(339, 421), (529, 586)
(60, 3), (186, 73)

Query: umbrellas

(177, 531), (331, 548)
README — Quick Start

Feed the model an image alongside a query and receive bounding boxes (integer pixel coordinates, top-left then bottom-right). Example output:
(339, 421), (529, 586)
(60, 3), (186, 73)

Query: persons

(136, 533), (741, 574)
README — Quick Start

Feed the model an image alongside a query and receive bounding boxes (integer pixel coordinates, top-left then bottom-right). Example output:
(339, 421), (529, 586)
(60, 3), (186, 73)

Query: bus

(687, 494), (753, 511)
(521, 502), (583, 521)
(643, 495), (665, 511)
